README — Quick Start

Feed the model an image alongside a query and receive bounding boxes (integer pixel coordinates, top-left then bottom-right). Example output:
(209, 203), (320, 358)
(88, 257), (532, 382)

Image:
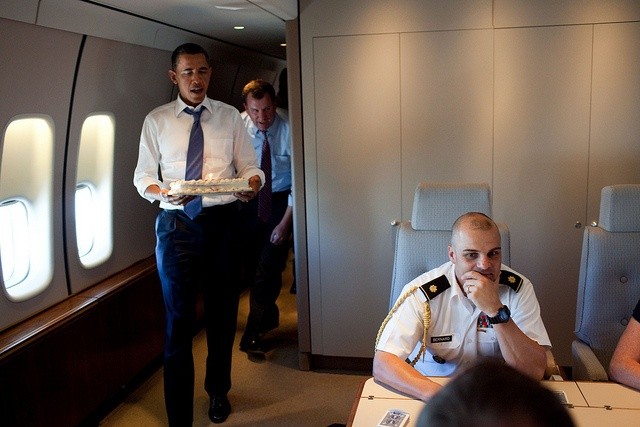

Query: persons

(232, 76), (292, 354)
(607, 299), (640, 391)
(415, 354), (578, 426)
(371, 210), (554, 403)
(131, 43), (268, 425)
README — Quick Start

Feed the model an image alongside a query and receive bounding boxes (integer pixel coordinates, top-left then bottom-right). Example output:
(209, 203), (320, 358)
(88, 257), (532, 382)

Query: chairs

(390, 179), (511, 376)
(570, 183), (639, 382)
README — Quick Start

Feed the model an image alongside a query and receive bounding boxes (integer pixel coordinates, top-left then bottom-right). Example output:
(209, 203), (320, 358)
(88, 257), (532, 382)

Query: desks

(348, 376), (640, 427)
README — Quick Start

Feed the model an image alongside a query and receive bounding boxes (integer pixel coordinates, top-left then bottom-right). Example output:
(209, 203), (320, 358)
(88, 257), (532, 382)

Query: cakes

(167, 179), (252, 196)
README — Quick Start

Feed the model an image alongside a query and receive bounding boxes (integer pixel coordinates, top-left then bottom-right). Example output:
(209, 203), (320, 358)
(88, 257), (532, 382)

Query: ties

(182, 106), (206, 220)
(259, 130), (273, 224)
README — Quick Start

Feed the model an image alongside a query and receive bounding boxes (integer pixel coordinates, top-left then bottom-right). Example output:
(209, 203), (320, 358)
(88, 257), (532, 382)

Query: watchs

(486, 304), (510, 323)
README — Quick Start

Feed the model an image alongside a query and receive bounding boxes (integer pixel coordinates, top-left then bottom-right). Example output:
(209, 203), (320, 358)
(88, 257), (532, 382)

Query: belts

(165, 209), (206, 216)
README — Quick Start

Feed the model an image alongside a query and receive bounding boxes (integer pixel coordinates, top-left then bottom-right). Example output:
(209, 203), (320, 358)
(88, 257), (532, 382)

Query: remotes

(378, 409), (410, 427)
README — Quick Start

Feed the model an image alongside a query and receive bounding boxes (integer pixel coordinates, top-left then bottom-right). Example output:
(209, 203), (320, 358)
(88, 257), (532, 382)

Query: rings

(467, 284), (470, 292)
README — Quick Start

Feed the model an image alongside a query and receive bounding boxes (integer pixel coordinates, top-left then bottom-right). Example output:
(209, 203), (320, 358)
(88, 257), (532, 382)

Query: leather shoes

(261, 318), (280, 334)
(239, 333), (266, 357)
(208, 393), (231, 423)
